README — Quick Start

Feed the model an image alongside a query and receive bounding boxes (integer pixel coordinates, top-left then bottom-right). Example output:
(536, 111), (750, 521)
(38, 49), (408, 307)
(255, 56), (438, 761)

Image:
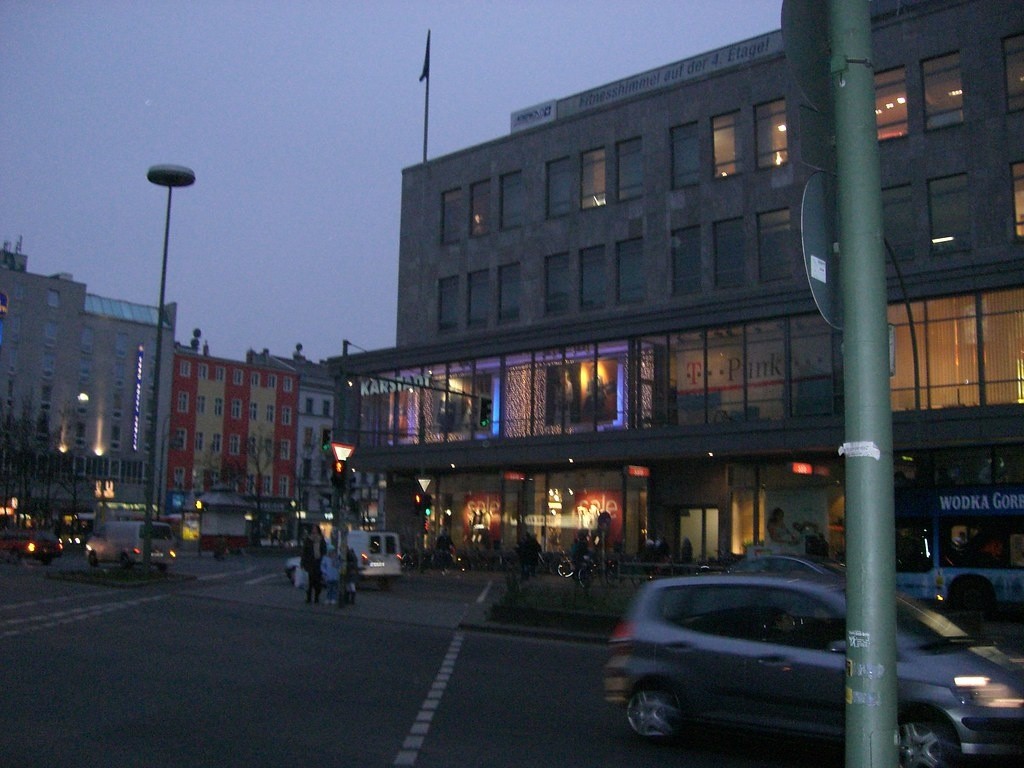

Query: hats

(326, 544), (335, 552)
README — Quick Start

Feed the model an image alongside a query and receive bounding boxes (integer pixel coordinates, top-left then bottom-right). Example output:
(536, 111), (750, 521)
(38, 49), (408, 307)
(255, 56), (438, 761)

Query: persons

(766, 508), (800, 556)
(301, 525), (358, 608)
(644, 533), (692, 575)
(436, 527), (456, 577)
(571, 533), (622, 583)
(516, 532), (542, 580)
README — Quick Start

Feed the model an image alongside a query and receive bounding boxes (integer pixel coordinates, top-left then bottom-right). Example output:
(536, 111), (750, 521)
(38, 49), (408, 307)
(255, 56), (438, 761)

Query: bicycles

(546, 557), (622, 587)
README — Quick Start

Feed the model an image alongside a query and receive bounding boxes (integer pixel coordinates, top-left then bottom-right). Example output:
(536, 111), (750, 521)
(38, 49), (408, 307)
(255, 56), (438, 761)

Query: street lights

(140, 162), (196, 577)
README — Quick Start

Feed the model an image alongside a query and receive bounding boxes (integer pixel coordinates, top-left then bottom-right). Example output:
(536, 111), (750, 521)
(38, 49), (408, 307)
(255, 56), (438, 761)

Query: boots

(344, 591), (349, 603)
(351, 590), (356, 605)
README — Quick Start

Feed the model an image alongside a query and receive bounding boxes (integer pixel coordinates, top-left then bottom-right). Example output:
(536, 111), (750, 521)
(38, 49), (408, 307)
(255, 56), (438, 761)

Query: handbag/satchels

(294, 567), (308, 587)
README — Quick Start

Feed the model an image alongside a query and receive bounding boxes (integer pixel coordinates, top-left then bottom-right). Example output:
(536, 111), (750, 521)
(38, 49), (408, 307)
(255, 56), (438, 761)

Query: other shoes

(324, 598), (329, 605)
(330, 598), (335, 605)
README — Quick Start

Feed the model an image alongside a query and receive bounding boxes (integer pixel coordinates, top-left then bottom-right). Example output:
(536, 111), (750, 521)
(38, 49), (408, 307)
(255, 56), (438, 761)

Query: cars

(0, 530), (65, 565)
(605, 552), (1024, 767)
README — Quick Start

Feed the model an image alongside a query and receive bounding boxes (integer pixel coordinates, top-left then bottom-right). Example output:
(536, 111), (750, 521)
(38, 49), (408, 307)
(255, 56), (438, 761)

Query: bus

(887, 482), (1024, 611)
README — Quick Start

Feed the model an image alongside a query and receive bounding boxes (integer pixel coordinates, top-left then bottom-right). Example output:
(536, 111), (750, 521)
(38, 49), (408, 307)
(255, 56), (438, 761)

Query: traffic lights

(321, 427), (332, 450)
(480, 395), (492, 431)
(333, 460), (344, 489)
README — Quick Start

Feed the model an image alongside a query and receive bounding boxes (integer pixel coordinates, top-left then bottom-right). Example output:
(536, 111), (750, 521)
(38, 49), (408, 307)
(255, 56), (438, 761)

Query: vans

(348, 530), (402, 577)
(86, 521), (177, 578)
